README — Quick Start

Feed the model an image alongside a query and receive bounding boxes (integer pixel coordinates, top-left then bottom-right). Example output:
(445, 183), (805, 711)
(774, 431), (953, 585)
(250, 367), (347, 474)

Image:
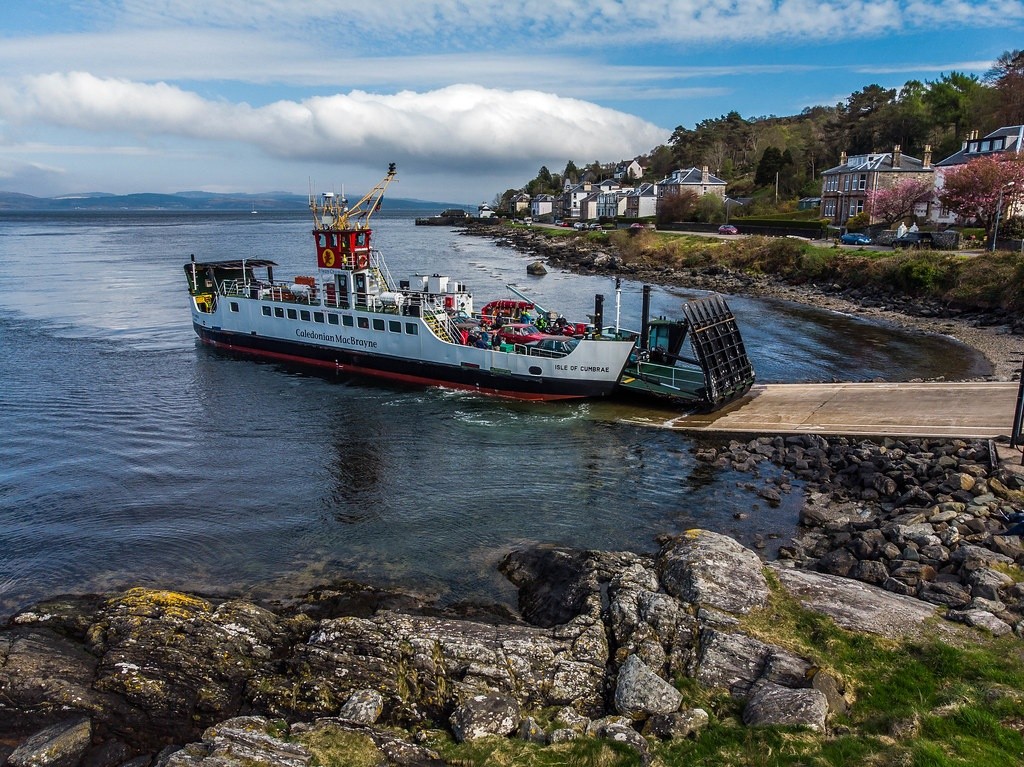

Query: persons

(249, 274), (258, 300)
(595, 312), (602, 335)
(460, 305), (573, 351)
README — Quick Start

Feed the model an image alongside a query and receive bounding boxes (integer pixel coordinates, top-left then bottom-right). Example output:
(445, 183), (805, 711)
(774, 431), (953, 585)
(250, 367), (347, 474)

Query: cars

(497, 324), (578, 357)
(841, 232), (871, 245)
(556, 220), (569, 226)
(630, 222), (644, 228)
(573, 221), (589, 230)
(718, 225), (739, 235)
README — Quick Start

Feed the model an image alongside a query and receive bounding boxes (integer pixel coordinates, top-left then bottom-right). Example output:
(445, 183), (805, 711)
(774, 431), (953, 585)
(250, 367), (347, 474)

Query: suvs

(590, 224), (602, 229)
(890, 230), (933, 248)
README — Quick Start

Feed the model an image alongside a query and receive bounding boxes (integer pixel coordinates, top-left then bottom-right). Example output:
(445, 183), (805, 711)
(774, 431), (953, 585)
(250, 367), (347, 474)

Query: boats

(183, 163), (642, 401)
(251, 211), (258, 213)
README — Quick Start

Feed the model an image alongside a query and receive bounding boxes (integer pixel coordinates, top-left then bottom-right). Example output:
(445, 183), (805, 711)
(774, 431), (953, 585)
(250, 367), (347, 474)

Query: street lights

(992, 181), (1016, 252)
